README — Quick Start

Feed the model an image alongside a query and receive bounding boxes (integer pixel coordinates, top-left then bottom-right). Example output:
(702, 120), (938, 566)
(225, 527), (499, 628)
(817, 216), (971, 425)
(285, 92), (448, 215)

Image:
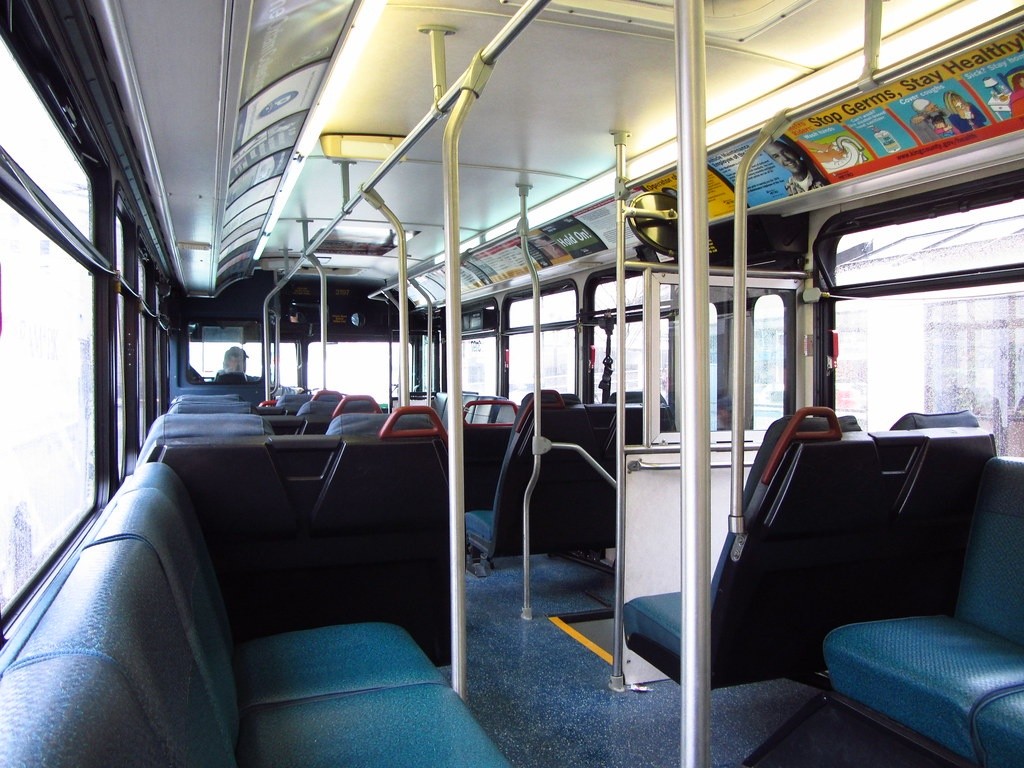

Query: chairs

(132, 386), (999, 694)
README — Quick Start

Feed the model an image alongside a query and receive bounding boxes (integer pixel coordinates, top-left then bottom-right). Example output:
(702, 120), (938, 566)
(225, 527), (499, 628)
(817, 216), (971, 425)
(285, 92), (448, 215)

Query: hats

(225, 347), (249, 358)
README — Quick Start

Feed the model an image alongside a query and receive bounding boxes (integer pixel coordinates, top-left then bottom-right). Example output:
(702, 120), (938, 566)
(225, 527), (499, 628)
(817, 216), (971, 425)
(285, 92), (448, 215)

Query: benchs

(0, 462), (519, 768)
(819, 453), (1024, 768)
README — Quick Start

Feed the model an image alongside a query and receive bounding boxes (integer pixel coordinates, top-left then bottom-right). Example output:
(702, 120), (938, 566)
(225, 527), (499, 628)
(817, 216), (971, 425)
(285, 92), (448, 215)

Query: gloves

(292, 387), (304, 395)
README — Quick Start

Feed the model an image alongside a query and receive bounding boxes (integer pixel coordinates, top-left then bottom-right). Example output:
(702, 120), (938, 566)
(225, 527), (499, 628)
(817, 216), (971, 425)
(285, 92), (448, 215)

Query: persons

(763, 142), (825, 197)
(216, 347), (304, 395)
